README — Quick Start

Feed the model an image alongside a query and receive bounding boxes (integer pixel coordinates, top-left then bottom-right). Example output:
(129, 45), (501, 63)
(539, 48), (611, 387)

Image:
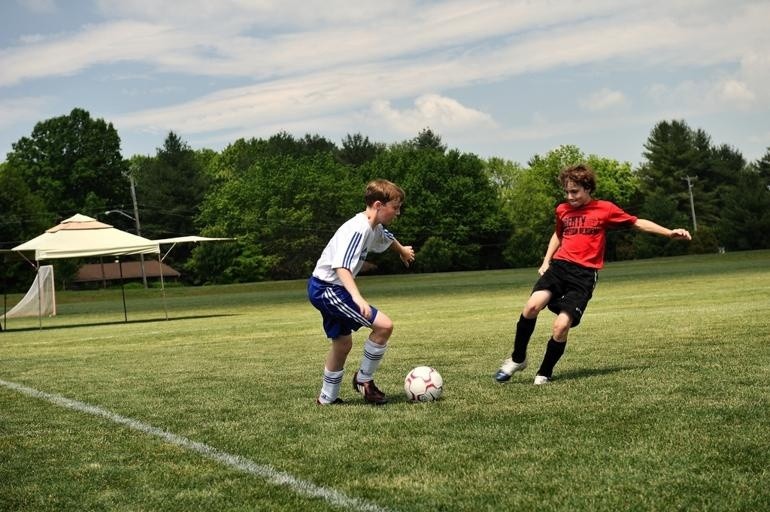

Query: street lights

(104, 210), (149, 289)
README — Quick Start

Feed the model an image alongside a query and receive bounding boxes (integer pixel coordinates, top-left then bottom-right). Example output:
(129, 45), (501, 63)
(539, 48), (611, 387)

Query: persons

(306, 179), (416, 407)
(495, 163), (693, 386)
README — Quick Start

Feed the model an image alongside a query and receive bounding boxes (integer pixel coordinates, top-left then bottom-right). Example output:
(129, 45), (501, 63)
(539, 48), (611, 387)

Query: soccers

(404, 366), (442, 401)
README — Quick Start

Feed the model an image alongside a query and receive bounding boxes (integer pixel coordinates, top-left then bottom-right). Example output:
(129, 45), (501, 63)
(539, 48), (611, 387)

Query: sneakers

(532, 370), (551, 386)
(495, 356), (528, 383)
(352, 372), (385, 403)
(316, 397), (350, 407)
